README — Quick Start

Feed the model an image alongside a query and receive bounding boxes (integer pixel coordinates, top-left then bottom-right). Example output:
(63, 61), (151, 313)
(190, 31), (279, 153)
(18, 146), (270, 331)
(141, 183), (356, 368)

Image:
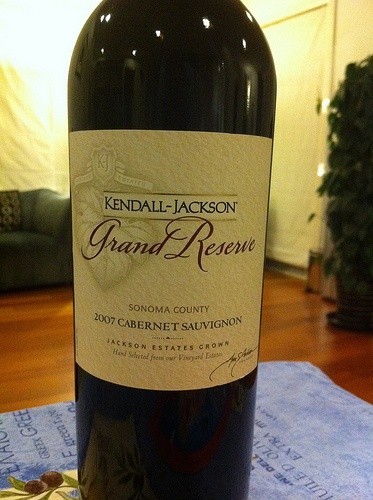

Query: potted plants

(306, 54), (373, 327)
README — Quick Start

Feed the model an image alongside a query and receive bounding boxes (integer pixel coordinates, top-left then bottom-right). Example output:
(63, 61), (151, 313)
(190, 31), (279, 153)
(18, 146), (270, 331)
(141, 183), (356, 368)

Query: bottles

(65, 0), (277, 500)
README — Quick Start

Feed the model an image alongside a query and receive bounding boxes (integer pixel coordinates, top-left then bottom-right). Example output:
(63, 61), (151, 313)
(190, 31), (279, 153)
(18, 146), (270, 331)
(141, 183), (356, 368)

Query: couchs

(0, 189), (72, 290)
